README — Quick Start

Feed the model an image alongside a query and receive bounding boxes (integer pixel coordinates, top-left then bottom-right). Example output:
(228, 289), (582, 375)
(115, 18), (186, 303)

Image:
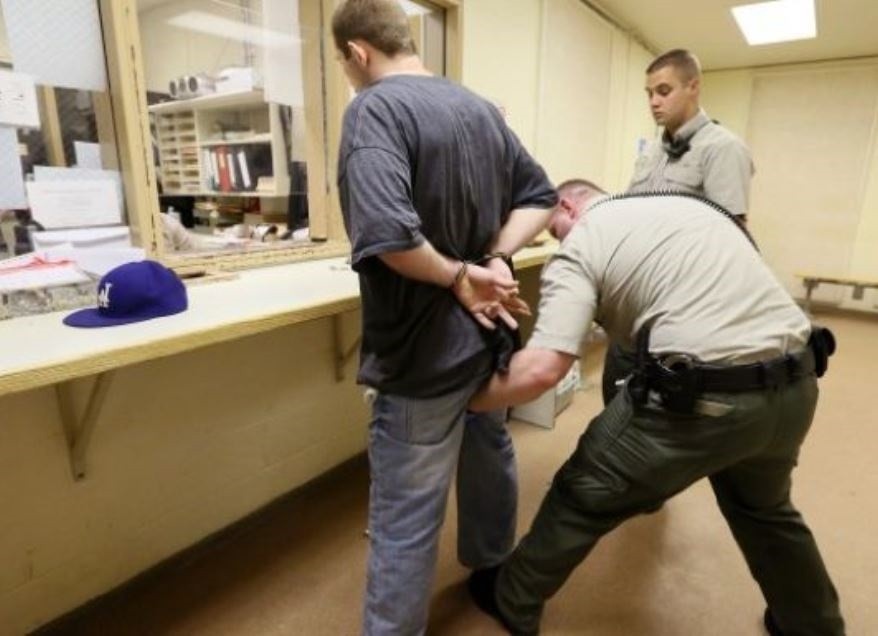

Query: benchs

(795, 271), (878, 307)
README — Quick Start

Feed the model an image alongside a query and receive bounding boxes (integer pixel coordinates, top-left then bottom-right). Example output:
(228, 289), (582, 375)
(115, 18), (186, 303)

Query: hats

(63, 260), (187, 327)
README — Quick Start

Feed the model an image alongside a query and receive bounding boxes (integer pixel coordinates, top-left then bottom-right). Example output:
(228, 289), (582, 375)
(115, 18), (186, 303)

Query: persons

(603, 49), (754, 514)
(467, 179), (845, 636)
(329, 0), (561, 636)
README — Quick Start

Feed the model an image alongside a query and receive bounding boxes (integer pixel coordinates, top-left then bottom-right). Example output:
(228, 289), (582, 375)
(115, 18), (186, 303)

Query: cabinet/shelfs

(149, 86), (292, 194)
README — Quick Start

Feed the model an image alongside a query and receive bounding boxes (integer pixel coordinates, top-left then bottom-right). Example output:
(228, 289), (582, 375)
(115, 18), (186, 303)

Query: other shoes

(469, 568), (538, 636)
(764, 607), (787, 636)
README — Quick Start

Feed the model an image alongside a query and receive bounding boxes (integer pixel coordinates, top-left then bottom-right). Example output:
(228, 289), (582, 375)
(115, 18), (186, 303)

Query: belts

(646, 348), (817, 390)
